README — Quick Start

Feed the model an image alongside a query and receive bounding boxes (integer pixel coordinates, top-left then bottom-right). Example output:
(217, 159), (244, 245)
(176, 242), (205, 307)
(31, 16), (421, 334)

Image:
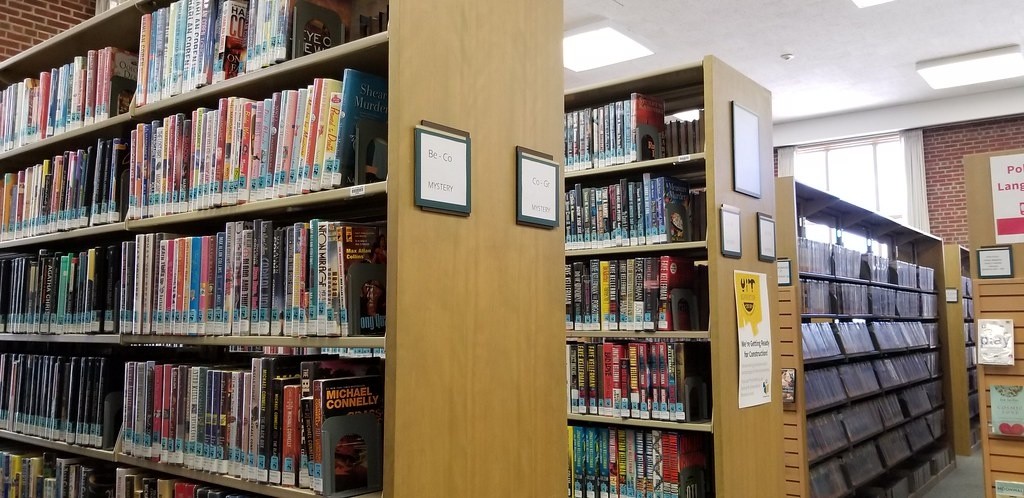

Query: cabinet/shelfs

(0, 0), (1024, 498)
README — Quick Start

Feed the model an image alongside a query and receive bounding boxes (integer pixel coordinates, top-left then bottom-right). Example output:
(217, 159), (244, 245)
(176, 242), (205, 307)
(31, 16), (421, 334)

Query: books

(564, 91), (705, 498)
(990, 383), (1024, 437)
(978, 318), (1015, 365)
(995, 480), (1024, 498)
(797, 238), (978, 498)
(0, 0), (388, 498)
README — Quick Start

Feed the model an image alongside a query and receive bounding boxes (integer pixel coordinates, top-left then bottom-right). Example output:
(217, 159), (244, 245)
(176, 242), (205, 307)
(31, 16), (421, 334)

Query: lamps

(563, 19), (658, 72)
(916, 44), (1024, 89)
